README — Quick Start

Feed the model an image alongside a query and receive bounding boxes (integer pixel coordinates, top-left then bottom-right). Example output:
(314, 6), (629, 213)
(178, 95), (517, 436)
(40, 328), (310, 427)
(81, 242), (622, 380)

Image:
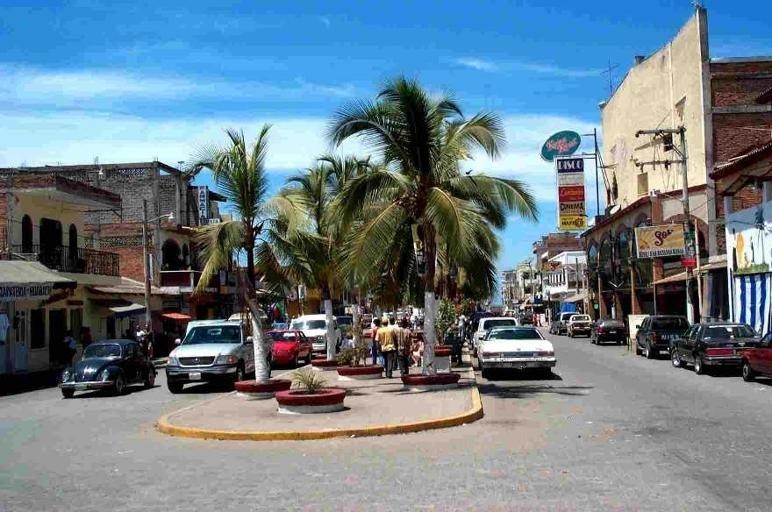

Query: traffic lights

(593, 299), (599, 310)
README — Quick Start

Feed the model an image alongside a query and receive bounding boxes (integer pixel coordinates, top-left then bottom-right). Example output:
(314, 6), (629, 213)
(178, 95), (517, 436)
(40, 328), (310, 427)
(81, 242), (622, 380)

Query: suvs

(634, 314), (690, 359)
(551, 312), (579, 335)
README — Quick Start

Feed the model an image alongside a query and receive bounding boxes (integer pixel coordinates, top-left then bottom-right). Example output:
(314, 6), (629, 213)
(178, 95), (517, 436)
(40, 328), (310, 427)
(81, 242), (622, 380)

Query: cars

(336, 308), (411, 339)
(503, 309), (515, 316)
(590, 318), (628, 345)
(477, 326), (557, 378)
(669, 322), (762, 375)
(59, 338), (159, 398)
(741, 330), (772, 382)
(565, 314), (593, 338)
(467, 312), (496, 340)
(264, 330), (314, 366)
(473, 317), (519, 357)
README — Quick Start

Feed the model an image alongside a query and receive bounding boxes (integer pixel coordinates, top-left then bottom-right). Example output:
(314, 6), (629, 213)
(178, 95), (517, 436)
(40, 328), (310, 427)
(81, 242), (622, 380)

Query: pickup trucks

(165, 320), (273, 393)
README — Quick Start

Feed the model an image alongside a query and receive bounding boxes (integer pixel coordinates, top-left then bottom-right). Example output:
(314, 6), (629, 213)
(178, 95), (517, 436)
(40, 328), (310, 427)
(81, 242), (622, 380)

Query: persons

(62, 329), (76, 364)
(371, 315), (424, 377)
(446, 313), (472, 367)
(131, 324), (153, 360)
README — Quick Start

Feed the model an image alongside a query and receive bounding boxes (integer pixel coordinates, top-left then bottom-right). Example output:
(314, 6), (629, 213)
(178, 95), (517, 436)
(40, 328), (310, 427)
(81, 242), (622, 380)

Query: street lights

(142, 211), (176, 330)
(648, 187), (696, 326)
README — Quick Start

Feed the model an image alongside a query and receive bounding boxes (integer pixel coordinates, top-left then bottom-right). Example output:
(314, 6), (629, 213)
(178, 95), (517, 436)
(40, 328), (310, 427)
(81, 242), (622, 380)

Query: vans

(284, 314), (342, 353)
(228, 311), (270, 320)
